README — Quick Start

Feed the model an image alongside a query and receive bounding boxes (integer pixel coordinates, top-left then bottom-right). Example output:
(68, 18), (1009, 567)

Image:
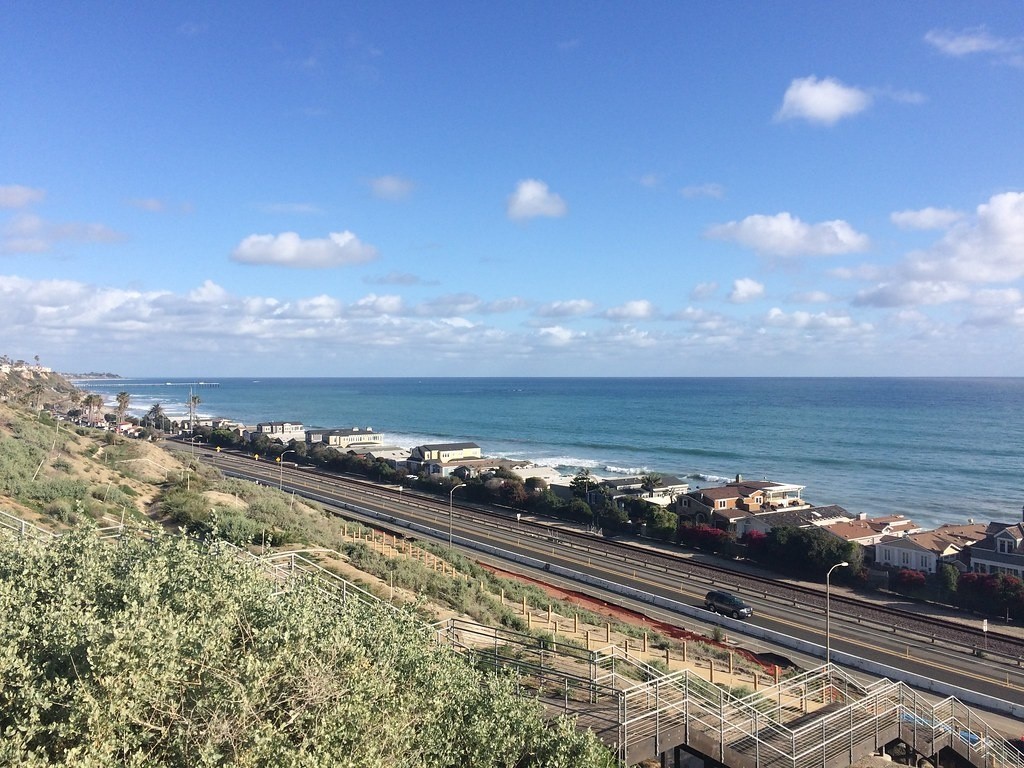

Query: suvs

(704, 590), (754, 619)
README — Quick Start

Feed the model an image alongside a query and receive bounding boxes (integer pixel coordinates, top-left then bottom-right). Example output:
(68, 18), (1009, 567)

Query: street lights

(278, 449), (296, 489)
(448, 483), (467, 548)
(824, 561), (849, 670)
(191, 435), (203, 459)
(516, 513), (522, 545)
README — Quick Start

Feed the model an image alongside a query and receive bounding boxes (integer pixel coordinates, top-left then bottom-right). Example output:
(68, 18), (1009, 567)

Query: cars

(41, 408), (84, 423)
